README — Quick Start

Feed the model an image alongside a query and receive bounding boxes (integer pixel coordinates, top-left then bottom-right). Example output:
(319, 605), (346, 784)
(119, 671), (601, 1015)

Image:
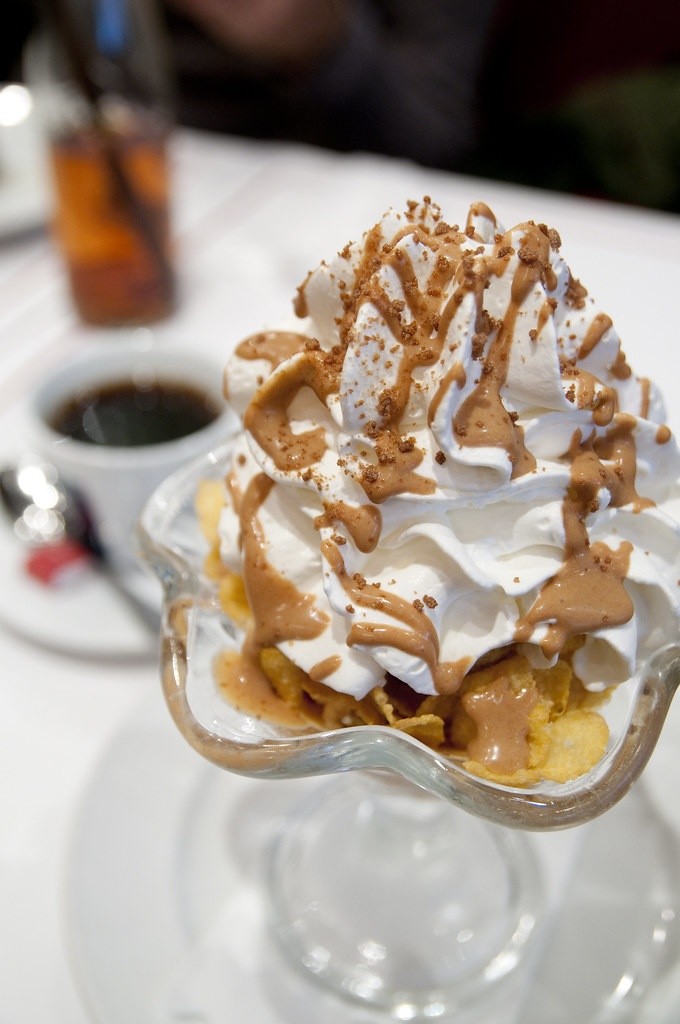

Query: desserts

(189, 195), (679, 783)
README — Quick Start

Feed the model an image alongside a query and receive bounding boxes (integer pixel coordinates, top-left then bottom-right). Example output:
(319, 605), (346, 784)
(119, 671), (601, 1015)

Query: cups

(38, 106), (187, 329)
(22, 351), (247, 562)
(138, 427), (680, 1022)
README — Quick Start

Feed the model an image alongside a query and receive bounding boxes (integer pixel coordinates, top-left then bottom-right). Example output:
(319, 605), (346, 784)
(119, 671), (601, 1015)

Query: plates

(2, 518), (174, 659)
